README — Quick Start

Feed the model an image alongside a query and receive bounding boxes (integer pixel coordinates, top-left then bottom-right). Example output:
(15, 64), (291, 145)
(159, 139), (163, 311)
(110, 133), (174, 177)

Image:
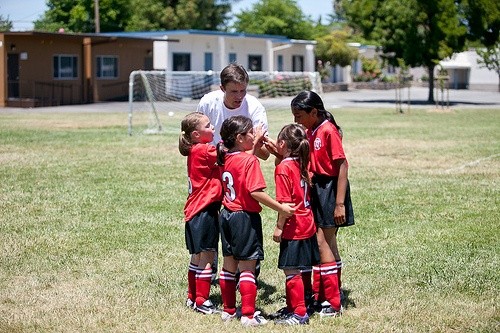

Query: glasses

(246, 130), (255, 136)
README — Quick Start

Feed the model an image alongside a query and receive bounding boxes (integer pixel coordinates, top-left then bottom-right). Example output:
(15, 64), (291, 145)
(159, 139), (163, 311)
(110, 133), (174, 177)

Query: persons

(178, 90), (355, 326)
(196, 64), (269, 289)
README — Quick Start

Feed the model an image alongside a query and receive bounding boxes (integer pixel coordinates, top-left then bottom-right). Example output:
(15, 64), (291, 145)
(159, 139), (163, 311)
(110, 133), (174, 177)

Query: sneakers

(186, 298), (194, 308)
(240, 311), (268, 326)
(193, 300), (220, 315)
(274, 313), (309, 325)
(306, 289), (344, 317)
(221, 309), (242, 321)
(268, 307), (292, 320)
